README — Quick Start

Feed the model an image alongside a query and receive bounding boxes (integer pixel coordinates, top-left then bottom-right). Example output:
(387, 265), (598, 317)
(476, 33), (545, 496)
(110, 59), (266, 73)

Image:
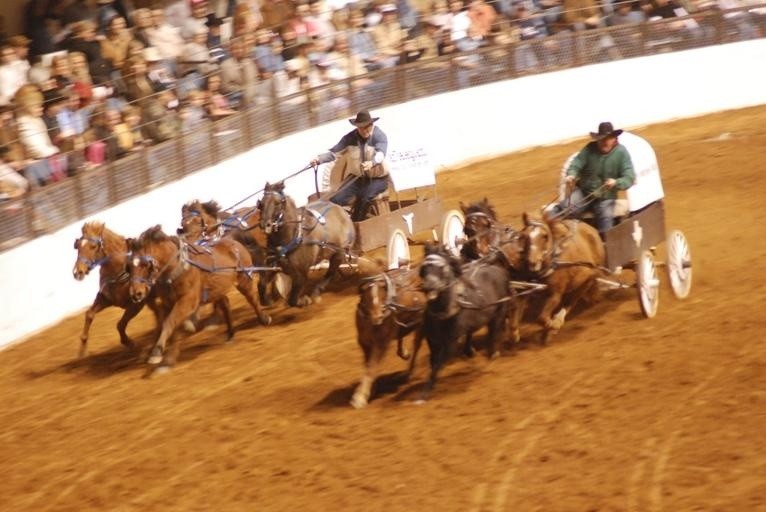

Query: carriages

(349, 132), (692, 412)
(71, 184), (440, 378)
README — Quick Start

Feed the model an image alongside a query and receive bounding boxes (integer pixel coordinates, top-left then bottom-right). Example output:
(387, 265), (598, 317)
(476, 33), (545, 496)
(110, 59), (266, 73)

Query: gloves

(360, 161), (374, 172)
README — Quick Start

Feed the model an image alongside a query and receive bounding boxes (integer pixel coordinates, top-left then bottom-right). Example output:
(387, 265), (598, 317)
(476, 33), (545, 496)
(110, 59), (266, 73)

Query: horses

(68, 179), (356, 367)
(345, 196), (607, 412)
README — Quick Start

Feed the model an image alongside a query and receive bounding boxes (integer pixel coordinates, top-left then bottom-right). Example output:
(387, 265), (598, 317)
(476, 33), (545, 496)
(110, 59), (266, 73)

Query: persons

(550, 122), (635, 230)
(311, 109), (388, 222)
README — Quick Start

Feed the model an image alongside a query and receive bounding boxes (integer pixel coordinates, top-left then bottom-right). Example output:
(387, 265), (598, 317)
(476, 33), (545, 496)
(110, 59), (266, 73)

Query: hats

(589, 122), (623, 141)
(348, 110), (379, 129)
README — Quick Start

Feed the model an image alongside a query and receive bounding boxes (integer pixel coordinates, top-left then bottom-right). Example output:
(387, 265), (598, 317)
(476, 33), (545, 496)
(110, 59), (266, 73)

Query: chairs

(305, 152), (392, 217)
(539, 151), (629, 223)
